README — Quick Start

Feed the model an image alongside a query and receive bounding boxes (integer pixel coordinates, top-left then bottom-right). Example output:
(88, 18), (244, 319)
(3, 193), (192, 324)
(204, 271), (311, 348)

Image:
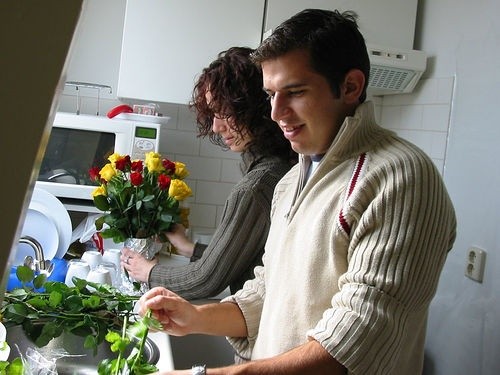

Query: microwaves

(33, 111), (161, 215)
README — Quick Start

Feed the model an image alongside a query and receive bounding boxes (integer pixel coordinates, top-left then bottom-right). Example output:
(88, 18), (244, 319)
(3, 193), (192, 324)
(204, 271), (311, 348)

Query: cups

(63, 248), (123, 292)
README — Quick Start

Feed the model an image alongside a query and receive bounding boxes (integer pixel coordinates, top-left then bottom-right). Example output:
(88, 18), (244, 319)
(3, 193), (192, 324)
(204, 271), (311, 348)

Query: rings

(127, 258), (129, 264)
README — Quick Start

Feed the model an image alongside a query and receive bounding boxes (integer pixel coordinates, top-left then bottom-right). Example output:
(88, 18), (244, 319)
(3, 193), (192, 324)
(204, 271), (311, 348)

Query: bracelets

(192, 365), (206, 375)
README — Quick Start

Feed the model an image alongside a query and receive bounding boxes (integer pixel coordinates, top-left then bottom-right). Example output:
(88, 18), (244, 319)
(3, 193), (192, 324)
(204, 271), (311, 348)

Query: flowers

(89, 150), (193, 243)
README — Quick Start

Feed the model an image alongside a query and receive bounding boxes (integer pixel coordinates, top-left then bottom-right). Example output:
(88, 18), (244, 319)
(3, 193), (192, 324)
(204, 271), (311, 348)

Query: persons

(133, 8), (457, 375)
(119, 47), (299, 301)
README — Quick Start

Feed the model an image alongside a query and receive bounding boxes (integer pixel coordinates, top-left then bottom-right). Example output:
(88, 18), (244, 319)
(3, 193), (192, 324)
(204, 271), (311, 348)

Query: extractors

(263, 30), (427, 97)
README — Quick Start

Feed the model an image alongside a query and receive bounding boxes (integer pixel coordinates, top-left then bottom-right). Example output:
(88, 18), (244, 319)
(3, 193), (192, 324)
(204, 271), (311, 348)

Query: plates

(13, 187), (72, 260)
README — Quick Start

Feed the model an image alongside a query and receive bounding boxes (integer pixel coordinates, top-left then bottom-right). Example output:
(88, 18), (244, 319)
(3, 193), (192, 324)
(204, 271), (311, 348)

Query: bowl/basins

(4, 318), (160, 375)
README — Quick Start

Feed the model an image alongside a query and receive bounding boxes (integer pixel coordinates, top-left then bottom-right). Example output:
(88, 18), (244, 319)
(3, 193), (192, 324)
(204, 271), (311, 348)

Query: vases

(116, 235), (163, 298)
